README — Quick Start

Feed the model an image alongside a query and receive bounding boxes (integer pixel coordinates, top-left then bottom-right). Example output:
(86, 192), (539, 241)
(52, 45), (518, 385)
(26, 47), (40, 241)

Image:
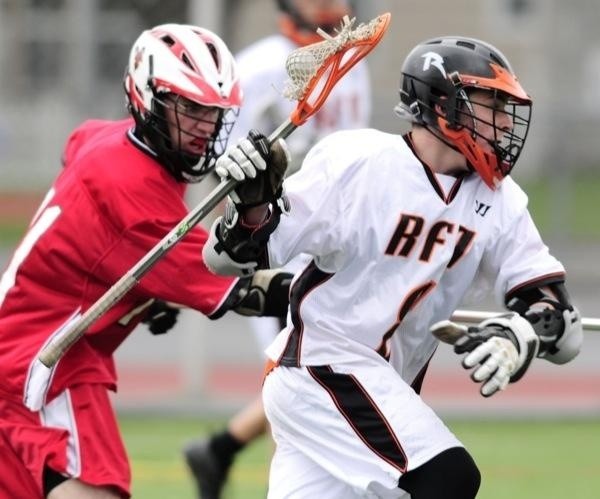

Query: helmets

(123, 24), (243, 184)
(395, 37), (531, 191)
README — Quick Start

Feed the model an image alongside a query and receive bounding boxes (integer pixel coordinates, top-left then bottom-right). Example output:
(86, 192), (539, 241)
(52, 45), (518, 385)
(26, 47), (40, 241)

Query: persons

(202, 35), (584, 498)
(185, 0), (371, 497)
(1, 21), (304, 499)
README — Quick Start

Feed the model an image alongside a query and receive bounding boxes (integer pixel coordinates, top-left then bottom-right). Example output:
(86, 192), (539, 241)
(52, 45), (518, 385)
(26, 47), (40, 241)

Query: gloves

(238, 268), (294, 317)
(217, 131), (292, 211)
(455, 313), (539, 397)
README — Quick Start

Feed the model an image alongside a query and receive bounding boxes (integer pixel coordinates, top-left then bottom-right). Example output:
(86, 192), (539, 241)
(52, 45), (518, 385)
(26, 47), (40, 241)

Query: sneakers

(186, 439), (225, 498)
